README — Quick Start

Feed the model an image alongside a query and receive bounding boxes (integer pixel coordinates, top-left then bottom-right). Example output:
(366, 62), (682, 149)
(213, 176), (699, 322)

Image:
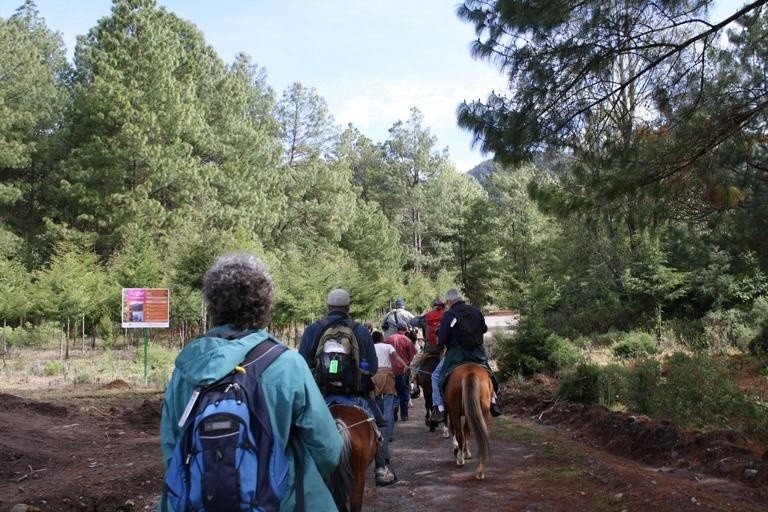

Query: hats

(327, 288), (350, 307)
(396, 298), (404, 306)
(396, 320), (407, 330)
(432, 300), (446, 309)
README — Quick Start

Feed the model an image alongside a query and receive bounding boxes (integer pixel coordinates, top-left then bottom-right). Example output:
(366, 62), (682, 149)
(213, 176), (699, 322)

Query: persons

(365, 330), (410, 442)
(292, 289), (398, 485)
(407, 300), (445, 400)
(157, 252), (352, 511)
(380, 298), (416, 339)
(427, 288), (502, 427)
(385, 325), (414, 421)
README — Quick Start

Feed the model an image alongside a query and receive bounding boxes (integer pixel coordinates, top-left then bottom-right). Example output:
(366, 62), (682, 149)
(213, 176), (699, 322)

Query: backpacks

(161, 337), (291, 512)
(448, 303), (483, 352)
(310, 317), (371, 398)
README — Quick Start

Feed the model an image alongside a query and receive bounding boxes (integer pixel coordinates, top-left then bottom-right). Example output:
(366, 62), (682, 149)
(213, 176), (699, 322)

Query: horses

(416, 357), (451, 438)
(328, 405), (379, 512)
(443, 363), (493, 480)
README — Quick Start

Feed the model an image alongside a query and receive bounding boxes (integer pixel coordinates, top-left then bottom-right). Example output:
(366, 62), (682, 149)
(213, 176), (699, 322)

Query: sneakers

(430, 408), (446, 422)
(374, 465), (395, 485)
(411, 387), (421, 398)
(490, 403), (504, 418)
